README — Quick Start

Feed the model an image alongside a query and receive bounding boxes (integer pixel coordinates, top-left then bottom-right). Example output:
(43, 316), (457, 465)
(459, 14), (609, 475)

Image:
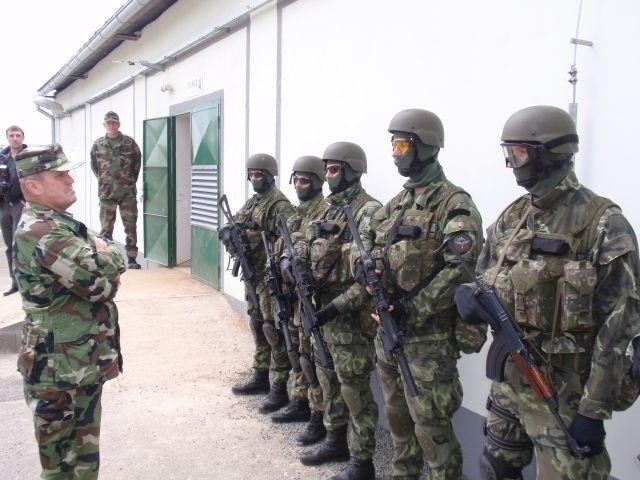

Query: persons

(452, 105), (639, 479)
(89, 109), (143, 272)
(1, 123), (38, 299)
(6, 143), (123, 478)
(222, 152), (298, 415)
(264, 155), (327, 443)
(350, 109), (487, 479)
(277, 142), (385, 479)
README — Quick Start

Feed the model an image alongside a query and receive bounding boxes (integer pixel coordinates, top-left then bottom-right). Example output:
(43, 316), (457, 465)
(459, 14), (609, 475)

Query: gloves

(566, 415), (605, 459)
(280, 258), (296, 286)
(219, 227), (236, 254)
(314, 302), (340, 327)
(454, 285), (494, 325)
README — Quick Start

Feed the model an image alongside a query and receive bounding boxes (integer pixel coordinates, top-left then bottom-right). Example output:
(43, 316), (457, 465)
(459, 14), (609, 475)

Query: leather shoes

(3, 288), (18, 295)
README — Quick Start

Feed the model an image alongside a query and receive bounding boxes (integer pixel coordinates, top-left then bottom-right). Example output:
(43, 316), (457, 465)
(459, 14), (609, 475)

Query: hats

(14, 144), (86, 178)
(103, 111), (119, 123)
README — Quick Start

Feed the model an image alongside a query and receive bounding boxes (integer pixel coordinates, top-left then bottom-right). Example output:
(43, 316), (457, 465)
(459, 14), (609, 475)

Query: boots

(296, 415), (375, 480)
(128, 258), (141, 269)
(232, 369), (311, 421)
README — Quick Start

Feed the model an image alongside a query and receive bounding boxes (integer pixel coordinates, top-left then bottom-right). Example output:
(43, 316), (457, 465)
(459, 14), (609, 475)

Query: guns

(434, 239), (592, 459)
(261, 230), (295, 353)
(217, 194), (261, 310)
(277, 218), (334, 368)
(343, 205), (419, 397)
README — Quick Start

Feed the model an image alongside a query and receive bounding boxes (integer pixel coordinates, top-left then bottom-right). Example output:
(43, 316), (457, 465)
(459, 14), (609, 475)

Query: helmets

(322, 142), (367, 172)
(388, 109), (444, 148)
(292, 157), (328, 181)
(502, 106), (579, 154)
(245, 154), (278, 175)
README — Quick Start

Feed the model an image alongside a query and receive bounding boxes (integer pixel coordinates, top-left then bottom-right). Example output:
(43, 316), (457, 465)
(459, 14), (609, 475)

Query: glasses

(392, 140), (413, 152)
(249, 173), (263, 178)
(500, 144), (543, 168)
(326, 166), (342, 174)
(294, 178), (311, 184)
